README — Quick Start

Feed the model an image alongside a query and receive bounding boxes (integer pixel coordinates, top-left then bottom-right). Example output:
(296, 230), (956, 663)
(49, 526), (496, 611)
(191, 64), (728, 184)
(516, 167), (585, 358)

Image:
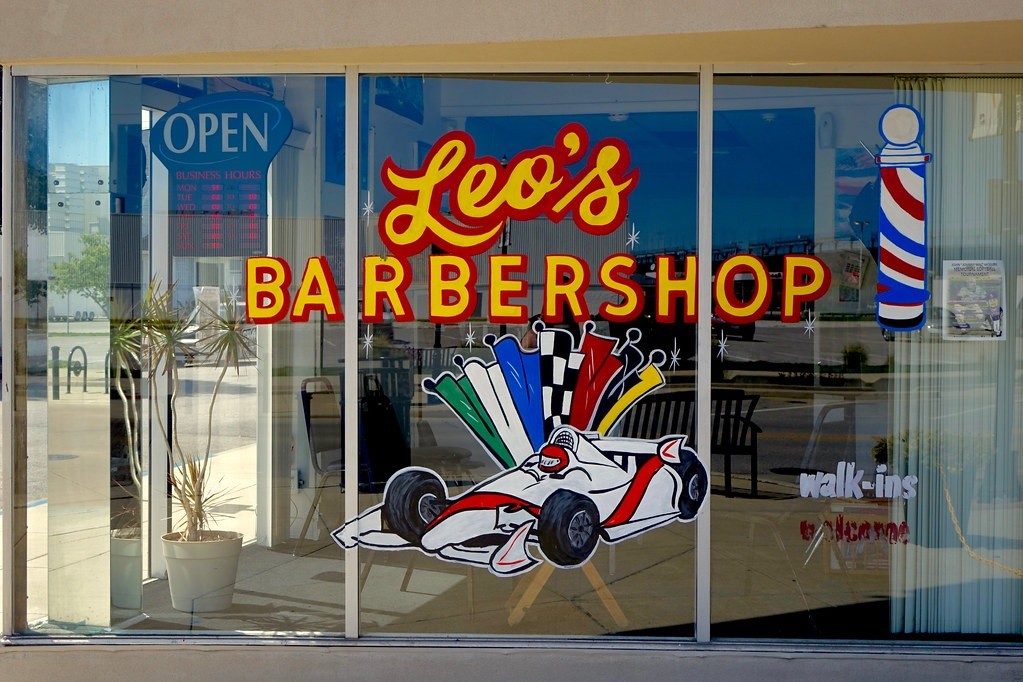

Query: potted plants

(111, 285), (142, 622)
(139, 288), (265, 616)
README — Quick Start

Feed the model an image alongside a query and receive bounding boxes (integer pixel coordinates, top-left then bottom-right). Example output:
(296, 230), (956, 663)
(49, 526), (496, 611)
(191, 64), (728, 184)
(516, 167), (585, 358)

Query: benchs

(627, 386), (763, 498)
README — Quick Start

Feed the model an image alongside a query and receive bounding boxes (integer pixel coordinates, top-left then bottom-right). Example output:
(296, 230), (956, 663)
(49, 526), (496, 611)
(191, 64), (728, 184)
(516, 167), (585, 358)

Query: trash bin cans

(337, 366), (412, 495)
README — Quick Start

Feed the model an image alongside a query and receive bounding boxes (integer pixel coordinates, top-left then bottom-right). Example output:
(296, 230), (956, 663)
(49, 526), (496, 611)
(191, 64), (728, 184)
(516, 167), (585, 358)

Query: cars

(171, 301), (256, 369)
(881, 307), (972, 343)
(710, 291), (756, 343)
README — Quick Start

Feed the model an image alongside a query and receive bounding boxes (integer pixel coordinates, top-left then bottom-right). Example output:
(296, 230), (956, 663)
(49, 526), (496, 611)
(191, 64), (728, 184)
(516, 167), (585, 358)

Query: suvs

(606, 279), (700, 367)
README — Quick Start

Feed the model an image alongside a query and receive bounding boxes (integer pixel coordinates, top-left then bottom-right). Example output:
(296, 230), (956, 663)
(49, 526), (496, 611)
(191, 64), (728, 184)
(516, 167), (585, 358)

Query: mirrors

(111, 76), (140, 631)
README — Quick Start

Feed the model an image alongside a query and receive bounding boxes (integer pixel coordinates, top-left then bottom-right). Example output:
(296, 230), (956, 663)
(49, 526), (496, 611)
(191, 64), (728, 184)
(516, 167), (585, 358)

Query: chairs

(295, 377), (483, 613)
